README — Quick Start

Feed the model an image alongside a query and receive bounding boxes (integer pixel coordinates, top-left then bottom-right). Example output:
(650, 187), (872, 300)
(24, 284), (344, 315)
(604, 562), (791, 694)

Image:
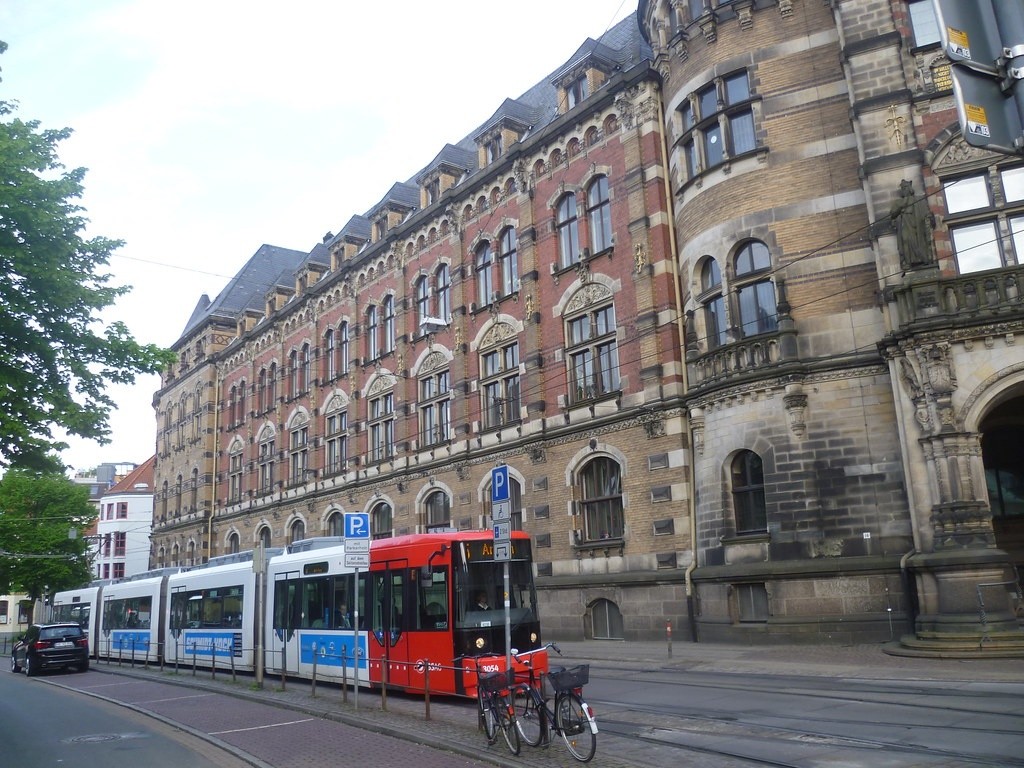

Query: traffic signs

(344, 512), (371, 539)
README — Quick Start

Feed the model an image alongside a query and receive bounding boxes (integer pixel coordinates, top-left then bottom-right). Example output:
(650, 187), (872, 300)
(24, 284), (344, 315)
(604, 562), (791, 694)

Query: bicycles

(510, 641), (599, 763)
(450, 651), (521, 756)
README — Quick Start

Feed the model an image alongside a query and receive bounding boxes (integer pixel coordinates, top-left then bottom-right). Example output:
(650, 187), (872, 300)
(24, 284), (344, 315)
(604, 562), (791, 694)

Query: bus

(47, 526), (550, 703)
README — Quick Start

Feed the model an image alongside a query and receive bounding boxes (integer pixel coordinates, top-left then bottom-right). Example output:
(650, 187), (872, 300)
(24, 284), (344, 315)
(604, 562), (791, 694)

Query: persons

(127, 612), (138, 628)
(473, 591), (492, 612)
(889, 179), (936, 269)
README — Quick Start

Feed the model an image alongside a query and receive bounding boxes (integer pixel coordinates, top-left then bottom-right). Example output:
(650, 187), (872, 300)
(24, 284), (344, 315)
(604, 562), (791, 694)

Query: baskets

(480, 668), (515, 692)
(548, 664), (589, 690)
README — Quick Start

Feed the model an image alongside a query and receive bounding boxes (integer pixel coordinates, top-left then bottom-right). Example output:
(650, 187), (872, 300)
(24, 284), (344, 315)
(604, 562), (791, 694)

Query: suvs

(10, 621), (91, 677)
